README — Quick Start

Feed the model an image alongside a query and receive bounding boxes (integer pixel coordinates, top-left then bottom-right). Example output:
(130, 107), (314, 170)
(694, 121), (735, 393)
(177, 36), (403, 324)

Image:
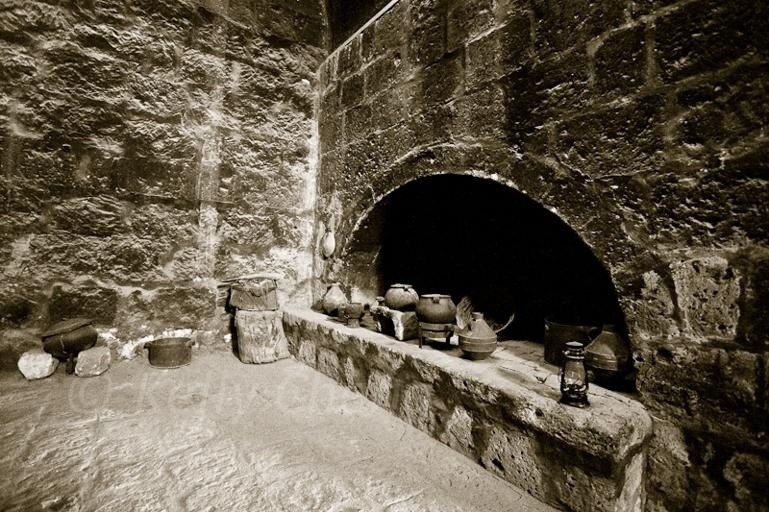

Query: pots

(416, 294), (456, 324)
(583, 328), (630, 384)
(144, 337), (196, 368)
(458, 313), (497, 361)
(544, 317), (598, 367)
(41, 317), (98, 360)
(385, 283), (419, 313)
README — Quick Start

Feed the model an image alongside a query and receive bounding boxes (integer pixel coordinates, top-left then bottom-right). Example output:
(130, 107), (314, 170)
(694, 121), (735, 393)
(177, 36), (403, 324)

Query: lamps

(558, 341), (590, 407)
